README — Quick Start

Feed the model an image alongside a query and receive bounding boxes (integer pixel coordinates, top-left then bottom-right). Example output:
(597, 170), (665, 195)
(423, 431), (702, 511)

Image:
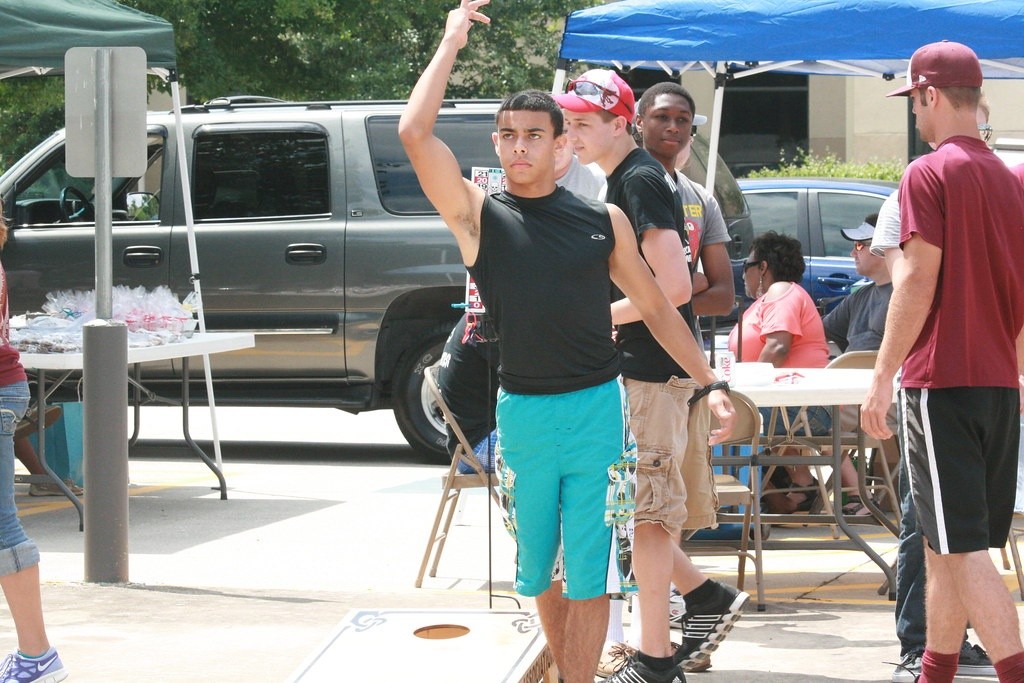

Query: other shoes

(594, 639), (640, 677)
(669, 584), (688, 629)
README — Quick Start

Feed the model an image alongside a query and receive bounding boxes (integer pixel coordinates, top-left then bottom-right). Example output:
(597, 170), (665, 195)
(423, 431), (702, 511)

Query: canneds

(714, 351), (736, 388)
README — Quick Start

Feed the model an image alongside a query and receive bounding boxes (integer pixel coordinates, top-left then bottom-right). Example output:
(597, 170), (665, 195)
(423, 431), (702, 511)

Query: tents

(560, 0), (1024, 195)
(0, 1), (227, 499)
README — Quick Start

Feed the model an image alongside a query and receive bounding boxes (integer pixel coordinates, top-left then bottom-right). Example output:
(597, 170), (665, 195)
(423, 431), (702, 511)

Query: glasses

(854, 240), (865, 251)
(979, 125), (993, 142)
(691, 126), (697, 136)
(563, 78), (633, 116)
(743, 259), (760, 272)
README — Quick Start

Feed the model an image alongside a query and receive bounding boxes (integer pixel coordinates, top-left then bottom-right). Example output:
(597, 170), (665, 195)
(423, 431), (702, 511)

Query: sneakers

(673, 581), (751, 671)
(892, 632), (993, 683)
(597, 650), (687, 683)
(0, 645), (68, 683)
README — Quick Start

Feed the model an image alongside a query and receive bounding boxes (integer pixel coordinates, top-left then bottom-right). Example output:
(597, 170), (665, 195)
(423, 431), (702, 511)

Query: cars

(736, 176), (900, 337)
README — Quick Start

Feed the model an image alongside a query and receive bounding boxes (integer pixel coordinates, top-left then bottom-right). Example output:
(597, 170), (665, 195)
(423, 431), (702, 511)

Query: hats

(840, 221), (875, 241)
(631, 98), (708, 127)
(552, 69), (636, 126)
(886, 40), (983, 97)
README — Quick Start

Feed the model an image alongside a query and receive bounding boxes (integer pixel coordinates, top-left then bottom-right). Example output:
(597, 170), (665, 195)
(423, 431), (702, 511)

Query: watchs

(686, 381), (731, 406)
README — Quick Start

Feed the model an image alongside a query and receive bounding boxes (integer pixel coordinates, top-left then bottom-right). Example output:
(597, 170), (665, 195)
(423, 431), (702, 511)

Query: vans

(0, 91), (757, 466)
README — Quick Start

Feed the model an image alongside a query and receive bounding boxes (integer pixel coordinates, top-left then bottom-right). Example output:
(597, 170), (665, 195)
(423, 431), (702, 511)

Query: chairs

(628, 351), (903, 611)
(416, 366), (499, 587)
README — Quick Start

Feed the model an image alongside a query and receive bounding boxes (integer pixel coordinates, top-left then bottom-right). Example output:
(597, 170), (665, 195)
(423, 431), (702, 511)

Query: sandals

(841, 495), (880, 516)
(785, 476), (827, 513)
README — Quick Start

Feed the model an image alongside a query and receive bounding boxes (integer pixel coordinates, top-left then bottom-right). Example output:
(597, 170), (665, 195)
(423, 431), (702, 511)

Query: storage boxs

(691, 414), (770, 540)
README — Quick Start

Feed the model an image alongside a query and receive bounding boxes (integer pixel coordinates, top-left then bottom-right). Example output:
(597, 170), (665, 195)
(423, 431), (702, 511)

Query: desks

(712, 367), (907, 601)
(18, 332), (255, 533)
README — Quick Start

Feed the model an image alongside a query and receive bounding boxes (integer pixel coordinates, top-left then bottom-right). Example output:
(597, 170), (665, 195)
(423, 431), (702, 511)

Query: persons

(729, 229), (829, 515)
(1, 259), (67, 683)
(395, 1), (624, 683)
(870, 97), (998, 683)
(553, 102), (609, 202)
(553, 69), (749, 683)
(821, 213), (893, 514)
(436, 313), (500, 474)
(1008, 163), (1024, 186)
(14, 405), (85, 495)
(635, 82), (735, 628)
(859, 39), (1024, 683)
(635, 99), (708, 171)
